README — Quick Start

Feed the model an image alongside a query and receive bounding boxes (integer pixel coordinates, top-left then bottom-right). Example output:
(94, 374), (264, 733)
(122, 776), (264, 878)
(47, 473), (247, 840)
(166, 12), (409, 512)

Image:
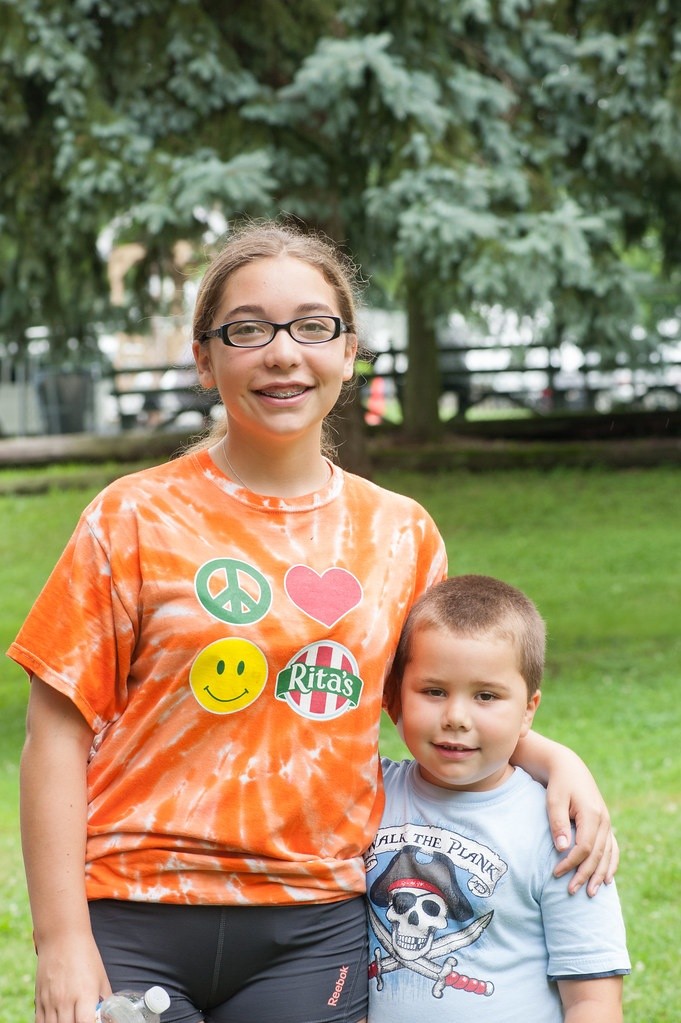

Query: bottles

(95, 986), (171, 1023)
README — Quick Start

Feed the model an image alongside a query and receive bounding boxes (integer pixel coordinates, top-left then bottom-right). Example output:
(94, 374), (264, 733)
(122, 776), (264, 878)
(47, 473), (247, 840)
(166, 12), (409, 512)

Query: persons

(89, 575), (632, 1023)
(9, 226), (622, 1023)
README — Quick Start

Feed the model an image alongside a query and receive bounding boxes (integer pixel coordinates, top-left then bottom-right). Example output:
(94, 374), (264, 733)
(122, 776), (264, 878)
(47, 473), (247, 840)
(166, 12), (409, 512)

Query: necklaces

(220, 440), (263, 498)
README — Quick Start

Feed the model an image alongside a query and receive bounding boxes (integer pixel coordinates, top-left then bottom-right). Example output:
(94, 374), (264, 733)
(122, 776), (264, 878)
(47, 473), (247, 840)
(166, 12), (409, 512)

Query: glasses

(198, 316), (349, 349)
